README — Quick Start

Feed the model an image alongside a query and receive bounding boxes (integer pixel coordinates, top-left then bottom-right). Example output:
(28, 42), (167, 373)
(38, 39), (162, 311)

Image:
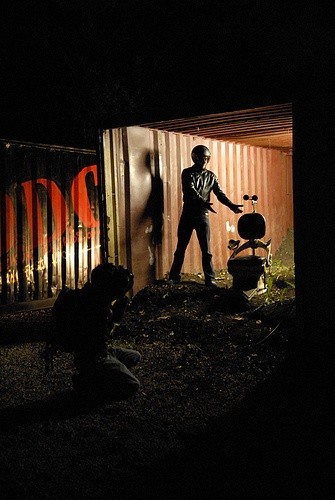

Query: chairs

(226, 195), (271, 291)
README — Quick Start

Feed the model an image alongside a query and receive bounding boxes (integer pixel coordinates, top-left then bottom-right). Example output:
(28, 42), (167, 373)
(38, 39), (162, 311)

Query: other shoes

(205, 280), (218, 289)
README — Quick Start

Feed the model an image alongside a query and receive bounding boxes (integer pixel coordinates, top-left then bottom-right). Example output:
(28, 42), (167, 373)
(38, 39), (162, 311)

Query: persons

(65, 263), (142, 404)
(169, 145), (243, 287)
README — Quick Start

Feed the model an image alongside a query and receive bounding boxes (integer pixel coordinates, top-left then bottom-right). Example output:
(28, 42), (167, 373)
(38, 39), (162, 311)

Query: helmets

(191, 145), (211, 164)
